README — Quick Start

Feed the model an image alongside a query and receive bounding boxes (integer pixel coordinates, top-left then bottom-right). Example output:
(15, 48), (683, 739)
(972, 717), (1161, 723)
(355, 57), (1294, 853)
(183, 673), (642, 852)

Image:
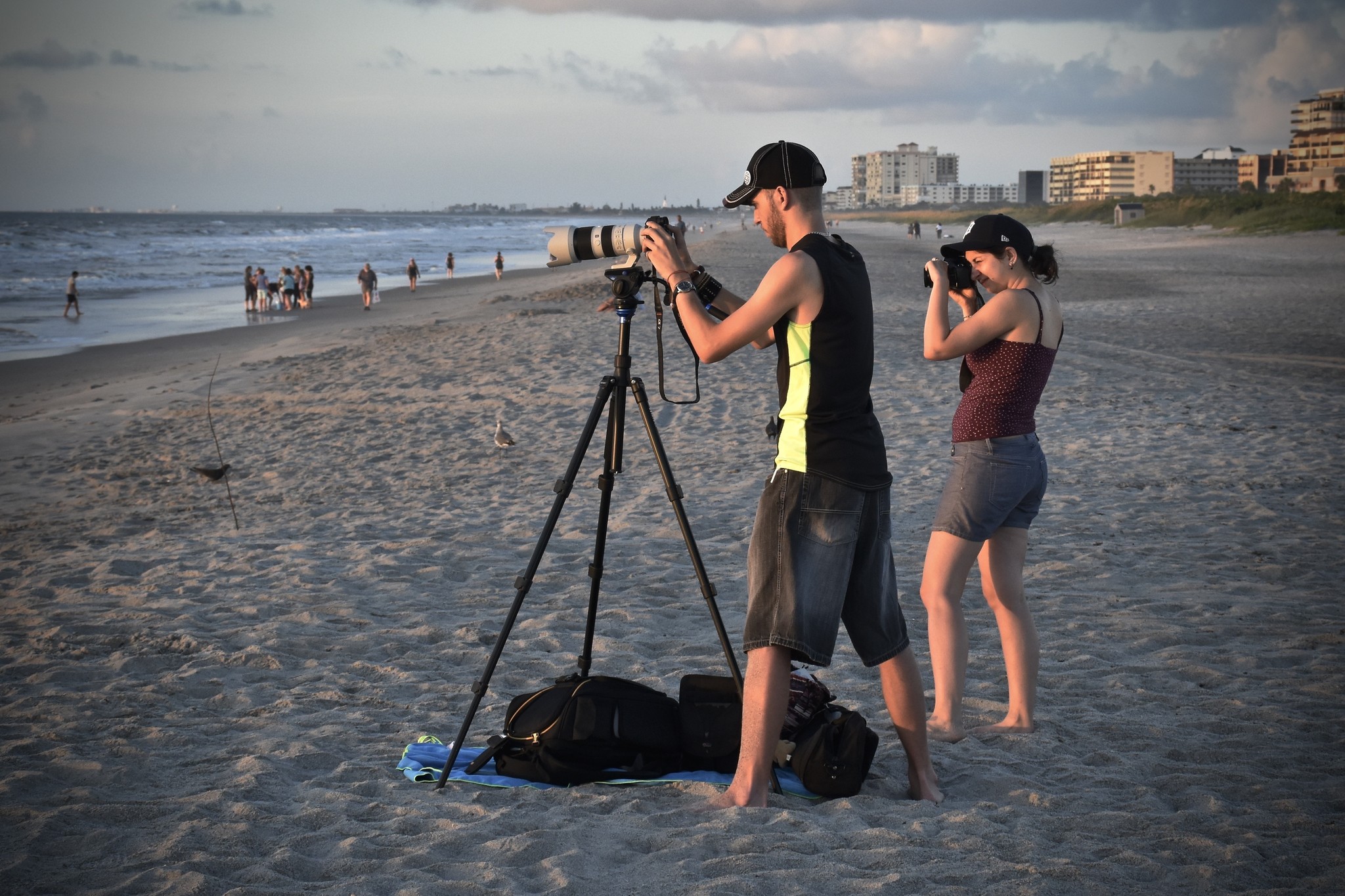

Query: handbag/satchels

(677, 675), (752, 773)
(786, 704), (879, 800)
(779, 663), (834, 743)
(465, 674), (686, 786)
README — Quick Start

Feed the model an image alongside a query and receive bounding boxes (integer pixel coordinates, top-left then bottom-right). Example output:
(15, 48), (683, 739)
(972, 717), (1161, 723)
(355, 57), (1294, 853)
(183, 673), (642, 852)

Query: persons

(64, 270), (84, 316)
(446, 253), (455, 279)
(406, 258), (420, 292)
(830, 218), (833, 227)
(913, 221), (922, 240)
(245, 264), (314, 312)
(494, 252), (504, 281)
(923, 212), (1105, 744)
(676, 215), (686, 239)
(357, 263), (378, 310)
(936, 222), (943, 238)
(835, 218), (840, 226)
(826, 219), (831, 228)
(640, 140), (947, 811)
(908, 223), (914, 238)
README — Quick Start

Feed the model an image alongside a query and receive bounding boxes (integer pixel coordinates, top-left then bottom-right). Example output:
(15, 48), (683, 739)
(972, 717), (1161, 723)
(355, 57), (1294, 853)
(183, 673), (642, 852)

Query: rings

(645, 251), (650, 258)
(934, 258), (939, 261)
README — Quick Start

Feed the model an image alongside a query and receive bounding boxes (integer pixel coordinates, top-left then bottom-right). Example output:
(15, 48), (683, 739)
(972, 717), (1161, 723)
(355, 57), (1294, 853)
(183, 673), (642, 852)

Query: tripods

(429, 266), (786, 796)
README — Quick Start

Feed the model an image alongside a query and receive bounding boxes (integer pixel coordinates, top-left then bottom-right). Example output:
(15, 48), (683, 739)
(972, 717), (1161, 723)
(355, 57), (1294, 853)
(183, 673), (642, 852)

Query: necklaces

(809, 231), (831, 236)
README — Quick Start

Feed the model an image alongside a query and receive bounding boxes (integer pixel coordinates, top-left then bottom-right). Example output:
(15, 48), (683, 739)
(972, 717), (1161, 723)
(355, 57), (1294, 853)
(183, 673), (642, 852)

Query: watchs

(671, 281), (697, 304)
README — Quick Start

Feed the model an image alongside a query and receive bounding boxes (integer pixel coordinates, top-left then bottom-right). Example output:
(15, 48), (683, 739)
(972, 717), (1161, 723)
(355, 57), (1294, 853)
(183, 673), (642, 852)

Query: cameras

(543, 216), (671, 268)
(923, 256), (971, 290)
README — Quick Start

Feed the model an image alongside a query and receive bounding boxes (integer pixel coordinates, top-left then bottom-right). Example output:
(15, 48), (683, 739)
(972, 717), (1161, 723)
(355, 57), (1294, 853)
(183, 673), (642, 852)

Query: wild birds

(494, 420), (516, 461)
(188, 464), (232, 484)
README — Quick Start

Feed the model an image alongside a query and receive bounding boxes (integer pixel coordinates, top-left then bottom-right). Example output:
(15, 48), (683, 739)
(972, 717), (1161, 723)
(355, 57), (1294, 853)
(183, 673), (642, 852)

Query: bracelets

(689, 265), (705, 282)
(667, 270), (689, 285)
(697, 272), (711, 292)
(964, 315), (973, 319)
(699, 276), (722, 303)
(674, 277), (691, 290)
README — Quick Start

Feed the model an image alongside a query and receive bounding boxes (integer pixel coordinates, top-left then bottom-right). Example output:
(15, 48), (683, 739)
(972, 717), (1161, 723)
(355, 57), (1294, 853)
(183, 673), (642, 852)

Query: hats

(940, 213), (1034, 258)
(722, 140), (827, 209)
